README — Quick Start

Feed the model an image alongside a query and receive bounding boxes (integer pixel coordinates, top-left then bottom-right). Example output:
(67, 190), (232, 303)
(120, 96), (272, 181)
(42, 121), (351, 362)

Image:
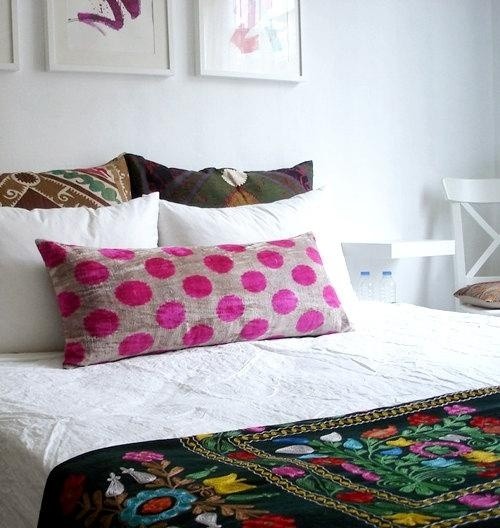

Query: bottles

(376, 271), (397, 303)
(357, 272), (374, 299)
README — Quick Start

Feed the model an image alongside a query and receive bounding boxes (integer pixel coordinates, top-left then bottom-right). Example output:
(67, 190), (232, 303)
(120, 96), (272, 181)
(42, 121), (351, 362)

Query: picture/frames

(41, 0), (177, 77)
(0, 0), (22, 70)
(195, 0), (305, 83)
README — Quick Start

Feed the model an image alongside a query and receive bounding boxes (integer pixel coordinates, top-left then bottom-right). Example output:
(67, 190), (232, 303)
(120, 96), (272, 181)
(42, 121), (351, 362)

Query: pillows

(452, 281), (500, 308)
(34, 231), (354, 371)
(1, 154), (131, 212)
(141, 190), (354, 306)
(1, 191), (159, 353)
(124, 152), (314, 210)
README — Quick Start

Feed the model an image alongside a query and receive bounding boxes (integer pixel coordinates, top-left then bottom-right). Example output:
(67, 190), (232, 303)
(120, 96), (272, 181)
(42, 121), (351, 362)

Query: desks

(339, 237), (458, 302)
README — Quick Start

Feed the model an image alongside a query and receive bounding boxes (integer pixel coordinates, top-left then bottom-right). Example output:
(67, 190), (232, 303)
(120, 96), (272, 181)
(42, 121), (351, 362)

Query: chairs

(442, 177), (499, 318)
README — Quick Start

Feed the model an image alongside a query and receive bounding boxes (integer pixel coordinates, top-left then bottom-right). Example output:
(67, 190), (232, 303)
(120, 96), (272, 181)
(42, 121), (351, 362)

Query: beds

(1, 305), (500, 528)
